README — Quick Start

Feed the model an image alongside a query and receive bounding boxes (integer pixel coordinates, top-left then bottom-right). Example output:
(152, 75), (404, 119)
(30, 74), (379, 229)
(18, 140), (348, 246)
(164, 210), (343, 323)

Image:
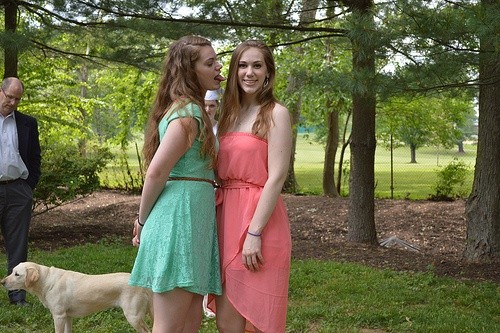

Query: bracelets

(137, 218), (145, 226)
(247, 231), (262, 237)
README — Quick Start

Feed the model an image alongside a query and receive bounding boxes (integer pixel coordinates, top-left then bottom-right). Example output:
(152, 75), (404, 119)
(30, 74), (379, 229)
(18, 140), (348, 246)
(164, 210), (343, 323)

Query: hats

(204, 89), (218, 100)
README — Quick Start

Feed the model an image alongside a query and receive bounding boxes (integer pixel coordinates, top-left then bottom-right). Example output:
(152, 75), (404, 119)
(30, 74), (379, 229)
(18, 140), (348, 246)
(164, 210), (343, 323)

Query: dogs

(1, 261), (154, 333)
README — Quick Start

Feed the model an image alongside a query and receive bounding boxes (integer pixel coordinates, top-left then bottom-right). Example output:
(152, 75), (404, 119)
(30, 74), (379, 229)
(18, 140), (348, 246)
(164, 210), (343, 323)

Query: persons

(125, 36), (229, 333)
(201, 89), (220, 151)
(0, 77), (41, 307)
(215, 39), (294, 333)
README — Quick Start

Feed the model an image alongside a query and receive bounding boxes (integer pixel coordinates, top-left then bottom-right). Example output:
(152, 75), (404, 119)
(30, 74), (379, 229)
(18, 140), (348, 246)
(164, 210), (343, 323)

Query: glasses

(2, 88), (21, 101)
